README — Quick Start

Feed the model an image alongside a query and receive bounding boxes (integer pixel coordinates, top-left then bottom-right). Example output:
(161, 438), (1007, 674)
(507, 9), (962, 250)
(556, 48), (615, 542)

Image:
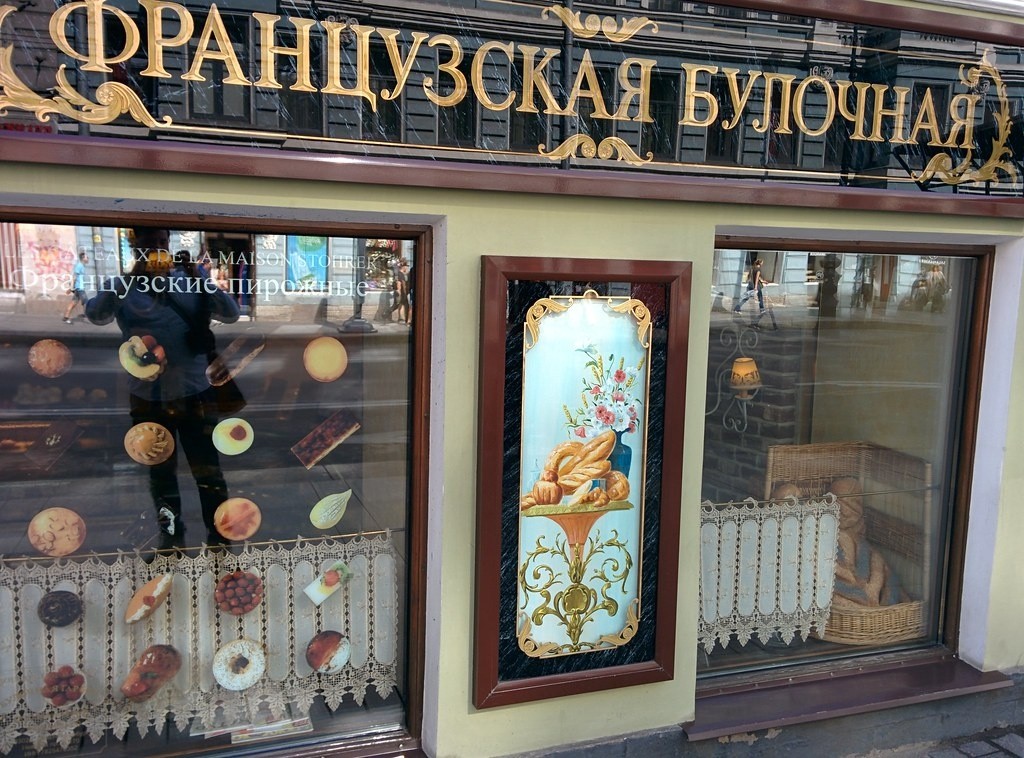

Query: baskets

(764, 440), (933, 647)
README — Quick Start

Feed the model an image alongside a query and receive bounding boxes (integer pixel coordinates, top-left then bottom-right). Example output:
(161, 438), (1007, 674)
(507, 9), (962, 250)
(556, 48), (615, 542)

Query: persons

(733, 258), (770, 314)
(62, 253), (91, 325)
(382, 260), (415, 327)
(85, 217), (241, 554)
(196, 253), (214, 280)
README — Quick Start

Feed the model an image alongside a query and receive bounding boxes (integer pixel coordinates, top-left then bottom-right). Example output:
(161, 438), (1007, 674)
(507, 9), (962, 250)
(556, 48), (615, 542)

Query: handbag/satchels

(207, 351), (248, 419)
(758, 280), (764, 290)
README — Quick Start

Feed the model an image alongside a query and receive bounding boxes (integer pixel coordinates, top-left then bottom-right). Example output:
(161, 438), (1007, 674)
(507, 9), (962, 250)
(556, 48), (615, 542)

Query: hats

(400, 260), (409, 266)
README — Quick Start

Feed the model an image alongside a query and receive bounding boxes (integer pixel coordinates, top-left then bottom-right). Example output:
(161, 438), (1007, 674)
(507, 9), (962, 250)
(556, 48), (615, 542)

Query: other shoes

(735, 310), (743, 315)
(62, 316), (73, 325)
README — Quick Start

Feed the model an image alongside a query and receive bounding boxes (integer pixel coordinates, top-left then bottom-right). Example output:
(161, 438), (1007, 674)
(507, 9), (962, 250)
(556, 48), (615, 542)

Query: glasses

(151, 238), (171, 246)
(84, 256), (89, 259)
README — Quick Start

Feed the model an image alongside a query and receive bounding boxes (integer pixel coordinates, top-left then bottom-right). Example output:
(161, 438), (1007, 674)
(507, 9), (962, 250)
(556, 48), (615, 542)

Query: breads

(122, 644), (182, 704)
(303, 337), (347, 382)
(769, 476), (912, 609)
(125, 573), (173, 623)
(27, 339), (73, 379)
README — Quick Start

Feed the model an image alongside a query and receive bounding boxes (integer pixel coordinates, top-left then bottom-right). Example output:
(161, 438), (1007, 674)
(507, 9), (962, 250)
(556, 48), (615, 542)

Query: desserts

(290, 408), (363, 471)
